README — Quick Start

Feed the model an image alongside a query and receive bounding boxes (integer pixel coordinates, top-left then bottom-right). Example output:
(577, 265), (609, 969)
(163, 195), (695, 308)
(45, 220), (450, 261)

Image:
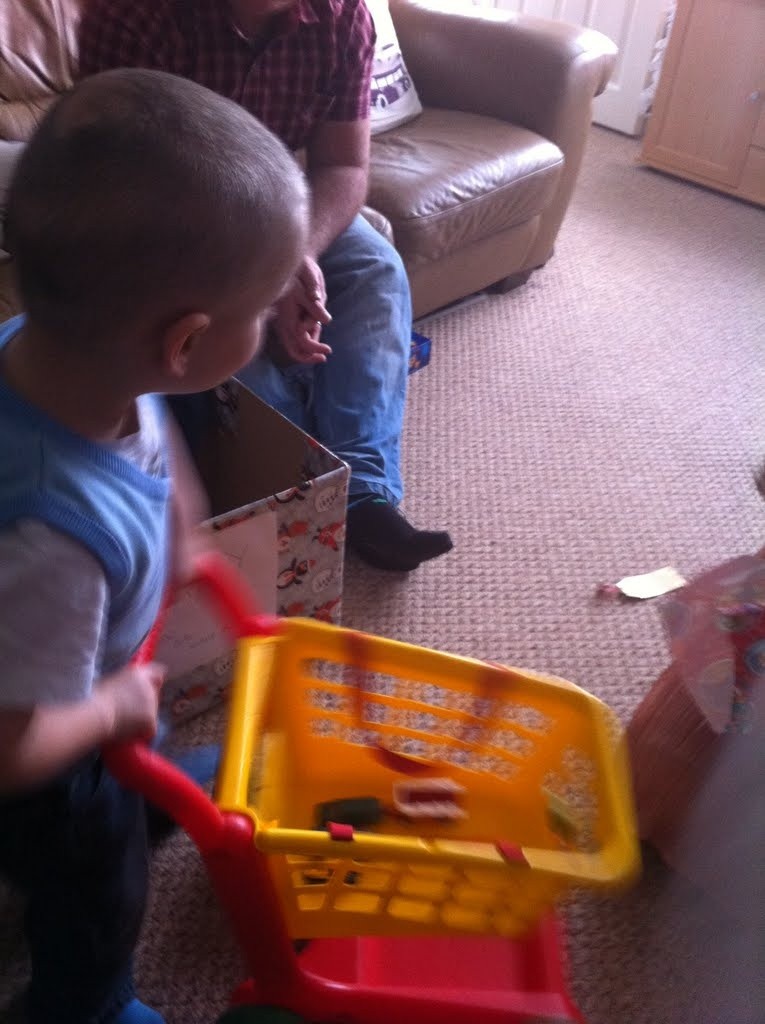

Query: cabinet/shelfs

(637, 0), (765, 210)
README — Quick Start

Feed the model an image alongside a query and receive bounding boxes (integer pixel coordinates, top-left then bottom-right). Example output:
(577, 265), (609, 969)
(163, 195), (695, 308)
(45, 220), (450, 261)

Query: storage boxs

(148, 375), (351, 734)
(408, 331), (432, 376)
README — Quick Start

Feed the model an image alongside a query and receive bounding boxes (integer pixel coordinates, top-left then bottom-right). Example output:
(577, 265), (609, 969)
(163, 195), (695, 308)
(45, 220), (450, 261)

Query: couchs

(0, 0), (620, 327)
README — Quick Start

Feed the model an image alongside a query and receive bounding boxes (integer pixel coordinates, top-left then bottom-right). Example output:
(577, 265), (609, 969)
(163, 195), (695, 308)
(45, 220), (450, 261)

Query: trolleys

(102, 550), (647, 1024)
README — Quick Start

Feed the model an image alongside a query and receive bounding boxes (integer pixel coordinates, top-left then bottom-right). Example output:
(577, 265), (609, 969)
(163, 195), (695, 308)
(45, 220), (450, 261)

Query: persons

(0, 73), (311, 1024)
(0, 0), (453, 572)
